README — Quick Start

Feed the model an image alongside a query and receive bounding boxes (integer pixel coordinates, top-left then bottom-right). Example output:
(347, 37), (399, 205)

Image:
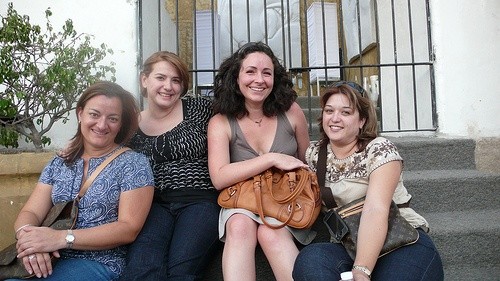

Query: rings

(28, 256), (36, 260)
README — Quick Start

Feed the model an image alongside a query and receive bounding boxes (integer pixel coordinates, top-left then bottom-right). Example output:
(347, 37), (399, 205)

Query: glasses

(237, 42), (271, 59)
(330, 81), (364, 98)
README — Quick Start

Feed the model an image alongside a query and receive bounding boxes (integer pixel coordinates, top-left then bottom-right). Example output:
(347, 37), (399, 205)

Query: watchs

(352, 265), (371, 278)
(65, 229), (75, 249)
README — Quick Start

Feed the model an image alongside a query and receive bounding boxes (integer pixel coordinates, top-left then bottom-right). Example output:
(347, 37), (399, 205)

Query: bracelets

(15, 224), (37, 240)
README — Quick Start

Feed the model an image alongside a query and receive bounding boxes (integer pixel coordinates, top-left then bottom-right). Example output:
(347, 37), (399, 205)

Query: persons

(206, 41), (318, 281)
(292, 80), (443, 281)
(0, 81), (155, 281)
(121, 51), (220, 281)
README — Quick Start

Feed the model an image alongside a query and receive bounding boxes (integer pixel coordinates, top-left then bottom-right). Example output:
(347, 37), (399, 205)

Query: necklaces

(83, 152), (104, 160)
(246, 116), (265, 127)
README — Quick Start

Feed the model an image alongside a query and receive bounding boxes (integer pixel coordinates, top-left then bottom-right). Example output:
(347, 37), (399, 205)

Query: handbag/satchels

(0, 199), (79, 280)
(217, 167), (323, 229)
(321, 196), (420, 262)
